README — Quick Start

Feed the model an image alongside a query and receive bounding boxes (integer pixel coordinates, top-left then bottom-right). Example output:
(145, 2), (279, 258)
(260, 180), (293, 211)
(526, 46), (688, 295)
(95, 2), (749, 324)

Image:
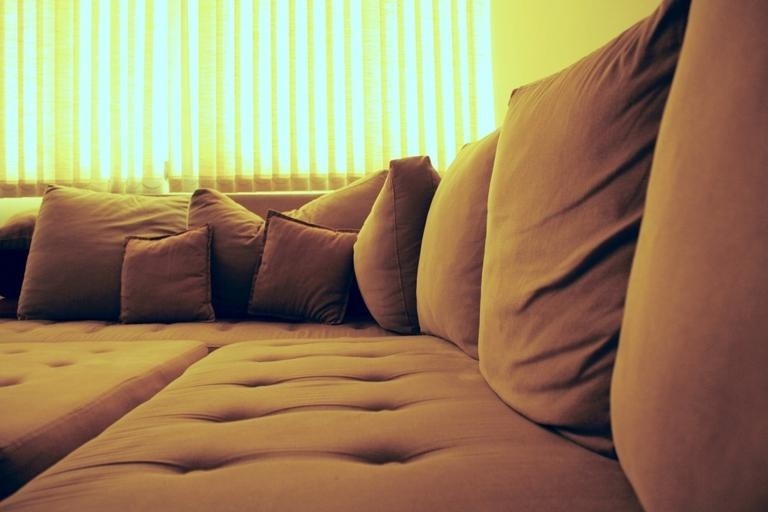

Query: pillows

(16, 155), (441, 334)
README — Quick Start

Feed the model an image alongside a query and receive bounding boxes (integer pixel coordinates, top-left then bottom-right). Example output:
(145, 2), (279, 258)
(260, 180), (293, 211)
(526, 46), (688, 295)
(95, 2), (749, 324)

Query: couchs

(3, 0), (768, 511)
